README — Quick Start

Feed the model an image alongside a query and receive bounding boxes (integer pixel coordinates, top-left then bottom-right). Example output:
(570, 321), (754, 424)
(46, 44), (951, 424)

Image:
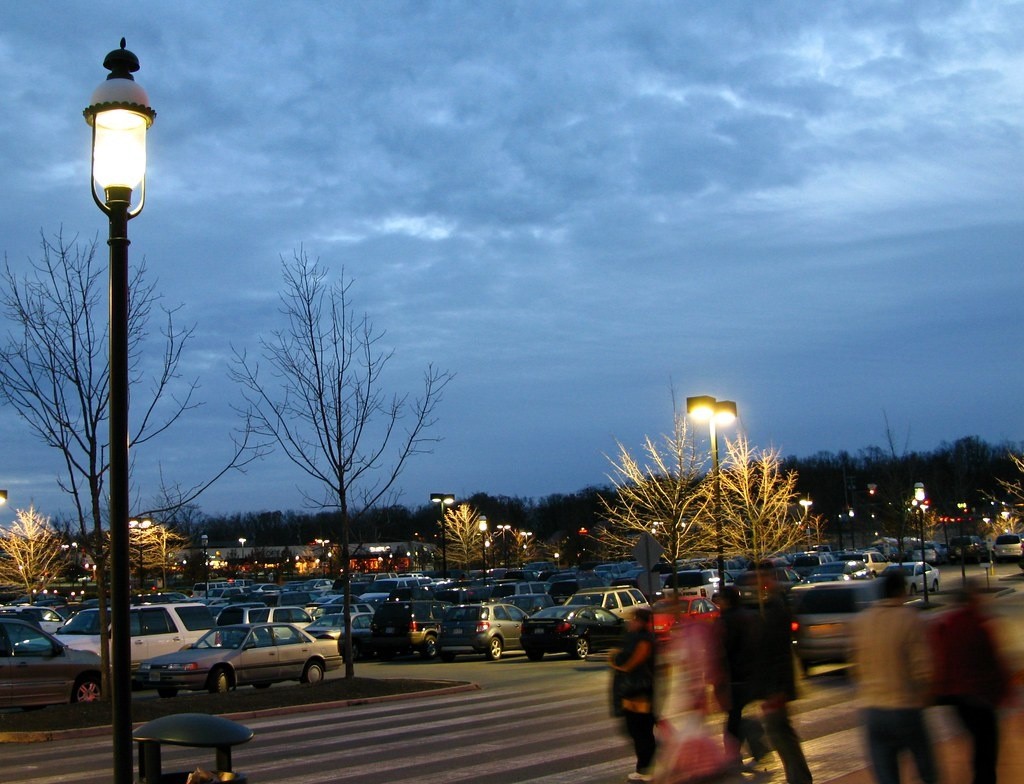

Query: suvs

(947, 536), (988, 564)
(17, 601), (222, 674)
(991, 534), (1024, 561)
(370, 600), (447, 659)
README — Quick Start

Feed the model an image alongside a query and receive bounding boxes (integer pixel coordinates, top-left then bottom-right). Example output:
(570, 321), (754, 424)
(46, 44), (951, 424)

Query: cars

(788, 581), (877, 679)
(0, 618), (101, 715)
(135, 623), (341, 694)
(0, 540), (946, 661)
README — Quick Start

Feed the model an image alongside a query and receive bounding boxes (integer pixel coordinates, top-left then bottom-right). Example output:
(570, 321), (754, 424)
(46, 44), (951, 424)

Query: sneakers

(628, 772), (652, 781)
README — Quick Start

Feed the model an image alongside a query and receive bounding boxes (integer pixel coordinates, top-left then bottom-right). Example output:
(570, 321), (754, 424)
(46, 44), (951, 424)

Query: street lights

(82, 35), (157, 784)
(480, 517), (487, 577)
(130, 519), (152, 593)
(686, 396), (738, 590)
(799, 500), (814, 551)
(430, 494), (456, 577)
(201, 535), (331, 594)
(914, 482), (930, 603)
(496, 524), (532, 569)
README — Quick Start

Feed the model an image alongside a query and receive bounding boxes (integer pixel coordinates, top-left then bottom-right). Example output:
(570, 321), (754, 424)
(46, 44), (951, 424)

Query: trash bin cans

(130, 713), (253, 784)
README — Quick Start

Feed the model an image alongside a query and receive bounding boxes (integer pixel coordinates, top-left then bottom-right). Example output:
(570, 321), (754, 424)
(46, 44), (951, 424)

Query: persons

(606, 560), (814, 784)
(848, 569), (1011, 784)
